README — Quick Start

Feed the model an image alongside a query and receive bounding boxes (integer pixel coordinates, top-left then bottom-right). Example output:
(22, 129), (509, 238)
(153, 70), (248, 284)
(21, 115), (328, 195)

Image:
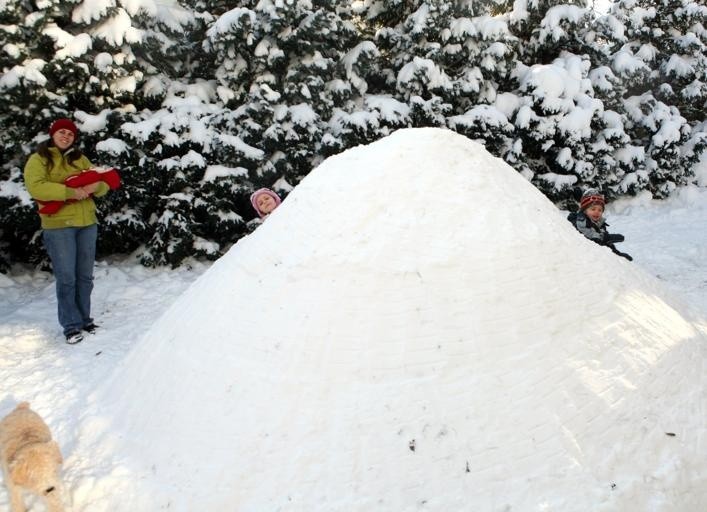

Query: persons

(567, 188), (634, 260)
(34, 164), (120, 214)
(24, 118), (110, 344)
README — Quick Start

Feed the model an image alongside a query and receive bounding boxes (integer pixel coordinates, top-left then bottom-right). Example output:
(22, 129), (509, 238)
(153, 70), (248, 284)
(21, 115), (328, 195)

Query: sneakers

(86, 324), (103, 334)
(65, 332), (83, 343)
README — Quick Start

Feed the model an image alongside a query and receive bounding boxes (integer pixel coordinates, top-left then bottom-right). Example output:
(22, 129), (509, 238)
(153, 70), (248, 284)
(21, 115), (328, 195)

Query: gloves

(615, 251), (633, 260)
(602, 234), (625, 244)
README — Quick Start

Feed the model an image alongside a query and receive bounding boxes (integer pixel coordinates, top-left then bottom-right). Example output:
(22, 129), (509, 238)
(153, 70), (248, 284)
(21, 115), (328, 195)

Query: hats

(50, 119), (76, 136)
(580, 188), (606, 209)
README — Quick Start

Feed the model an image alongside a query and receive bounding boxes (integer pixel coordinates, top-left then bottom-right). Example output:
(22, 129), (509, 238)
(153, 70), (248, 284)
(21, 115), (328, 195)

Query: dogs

(0, 400), (66, 512)
